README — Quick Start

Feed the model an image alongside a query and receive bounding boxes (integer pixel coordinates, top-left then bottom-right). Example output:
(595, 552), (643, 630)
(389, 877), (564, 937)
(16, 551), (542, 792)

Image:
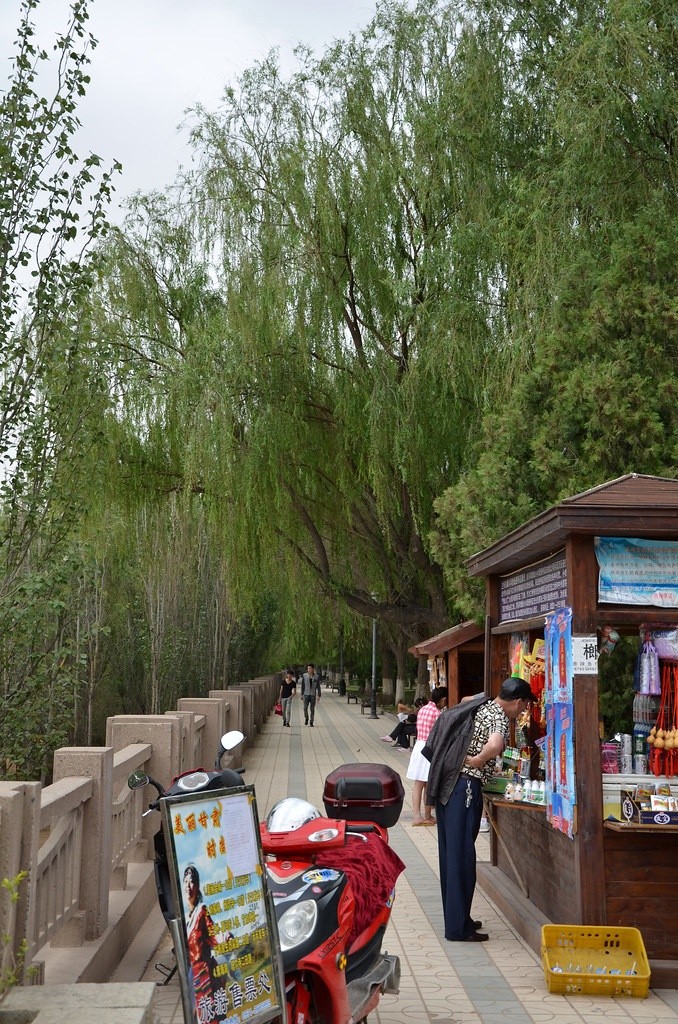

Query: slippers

(412, 819), (434, 826)
(426, 816), (437, 824)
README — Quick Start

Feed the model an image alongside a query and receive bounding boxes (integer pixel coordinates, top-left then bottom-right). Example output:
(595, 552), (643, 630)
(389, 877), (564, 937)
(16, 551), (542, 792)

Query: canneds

(518, 759), (531, 776)
(635, 754), (647, 774)
(518, 775), (529, 787)
(620, 734), (632, 754)
(635, 734), (646, 753)
(621, 755), (632, 773)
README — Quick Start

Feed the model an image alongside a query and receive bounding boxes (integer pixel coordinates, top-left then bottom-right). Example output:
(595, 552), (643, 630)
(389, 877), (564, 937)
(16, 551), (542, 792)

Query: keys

(466, 780), (472, 808)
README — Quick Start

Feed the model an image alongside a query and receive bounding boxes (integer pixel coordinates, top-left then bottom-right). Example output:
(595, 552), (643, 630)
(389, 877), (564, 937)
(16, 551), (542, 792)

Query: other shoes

(286, 723), (290, 727)
(283, 721), (286, 726)
(310, 723), (313, 726)
(305, 719), (308, 725)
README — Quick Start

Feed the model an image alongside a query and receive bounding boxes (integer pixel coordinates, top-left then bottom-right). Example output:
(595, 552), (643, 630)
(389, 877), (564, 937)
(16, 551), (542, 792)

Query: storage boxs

(637, 811), (678, 825)
(541, 924), (651, 998)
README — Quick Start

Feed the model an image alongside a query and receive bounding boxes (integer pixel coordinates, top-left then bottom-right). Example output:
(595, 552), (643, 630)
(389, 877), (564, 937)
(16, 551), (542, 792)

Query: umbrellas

(640, 641), (661, 695)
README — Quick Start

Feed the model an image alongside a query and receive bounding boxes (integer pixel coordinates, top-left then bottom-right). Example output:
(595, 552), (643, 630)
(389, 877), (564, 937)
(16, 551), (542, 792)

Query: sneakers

(390, 741), (401, 748)
(381, 736), (393, 742)
(398, 747), (410, 752)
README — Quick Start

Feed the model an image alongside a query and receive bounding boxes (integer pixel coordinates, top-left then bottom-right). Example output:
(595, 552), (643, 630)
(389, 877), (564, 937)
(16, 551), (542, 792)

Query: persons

(421, 678), (538, 942)
(182, 862), (236, 1024)
(381, 697), (429, 749)
(407, 687), (448, 826)
(301, 664), (327, 727)
(279, 671), (300, 727)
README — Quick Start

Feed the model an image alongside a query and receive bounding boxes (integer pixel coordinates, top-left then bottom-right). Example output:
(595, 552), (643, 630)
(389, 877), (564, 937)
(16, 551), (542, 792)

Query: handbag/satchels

(275, 705), (283, 716)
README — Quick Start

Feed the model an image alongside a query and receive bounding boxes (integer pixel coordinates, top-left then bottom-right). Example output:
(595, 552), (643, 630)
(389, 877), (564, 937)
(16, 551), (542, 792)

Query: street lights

(371, 590), (380, 720)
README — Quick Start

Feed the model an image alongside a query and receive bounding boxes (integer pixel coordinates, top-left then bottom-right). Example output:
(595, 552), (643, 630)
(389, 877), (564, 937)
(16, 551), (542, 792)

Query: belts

(459, 772), (481, 783)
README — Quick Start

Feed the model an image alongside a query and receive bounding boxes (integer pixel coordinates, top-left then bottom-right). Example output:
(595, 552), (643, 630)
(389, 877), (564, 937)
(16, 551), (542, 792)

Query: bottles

(522, 780), (531, 802)
(504, 783), (514, 800)
(503, 746), (520, 765)
(531, 780), (540, 803)
(514, 784), (521, 800)
(540, 781), (546, 804)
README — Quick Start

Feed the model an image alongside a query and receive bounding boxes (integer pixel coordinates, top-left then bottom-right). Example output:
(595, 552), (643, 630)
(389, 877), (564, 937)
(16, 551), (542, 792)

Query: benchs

(326, 681), (340, 693)
(348, 690), (359, 704)
(361, 698), (371, 715)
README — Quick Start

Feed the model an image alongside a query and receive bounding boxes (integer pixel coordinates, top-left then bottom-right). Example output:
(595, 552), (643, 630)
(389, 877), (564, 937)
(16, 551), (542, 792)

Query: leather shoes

(457, 933), (489, 941)
(473, 921), (482, 930)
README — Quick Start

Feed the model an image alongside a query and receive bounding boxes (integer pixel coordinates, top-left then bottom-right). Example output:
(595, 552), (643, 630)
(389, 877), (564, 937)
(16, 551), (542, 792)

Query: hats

(501, 677), (538, 702)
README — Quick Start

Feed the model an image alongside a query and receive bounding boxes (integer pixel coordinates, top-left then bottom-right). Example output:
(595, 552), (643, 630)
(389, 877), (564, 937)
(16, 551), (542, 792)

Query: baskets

(542, 924), (651, 999)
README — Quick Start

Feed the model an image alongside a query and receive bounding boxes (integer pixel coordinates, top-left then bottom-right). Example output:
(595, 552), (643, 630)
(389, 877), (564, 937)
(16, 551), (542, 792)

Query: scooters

(131, 729), (247, 985)
(249, 763), (405, 1024)
